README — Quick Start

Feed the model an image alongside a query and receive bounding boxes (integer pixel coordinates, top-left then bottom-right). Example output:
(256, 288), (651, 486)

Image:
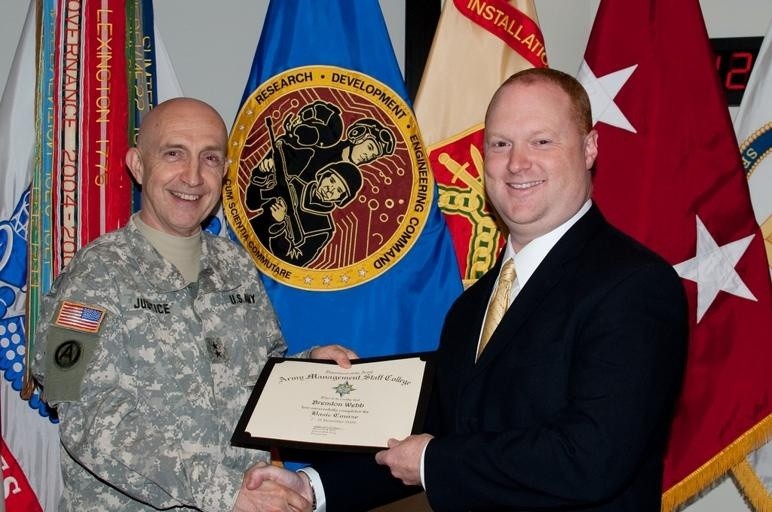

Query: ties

(476, 256), (517, 364)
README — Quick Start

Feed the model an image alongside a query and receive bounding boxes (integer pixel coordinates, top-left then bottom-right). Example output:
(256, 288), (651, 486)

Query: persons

(249, 64), (692, 511)
(23, 93), (316, 510)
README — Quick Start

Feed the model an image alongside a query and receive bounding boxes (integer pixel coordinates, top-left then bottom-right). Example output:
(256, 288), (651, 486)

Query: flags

(719, 28), (771, 511)
(0, 1), (189, 510)
(405, 2), (564, 299)
(567, 1), (768, 510)
(201, 1), (462, 357)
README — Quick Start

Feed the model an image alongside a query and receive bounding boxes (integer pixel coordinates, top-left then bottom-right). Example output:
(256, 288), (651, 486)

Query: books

(238, 356), (425, 447)
